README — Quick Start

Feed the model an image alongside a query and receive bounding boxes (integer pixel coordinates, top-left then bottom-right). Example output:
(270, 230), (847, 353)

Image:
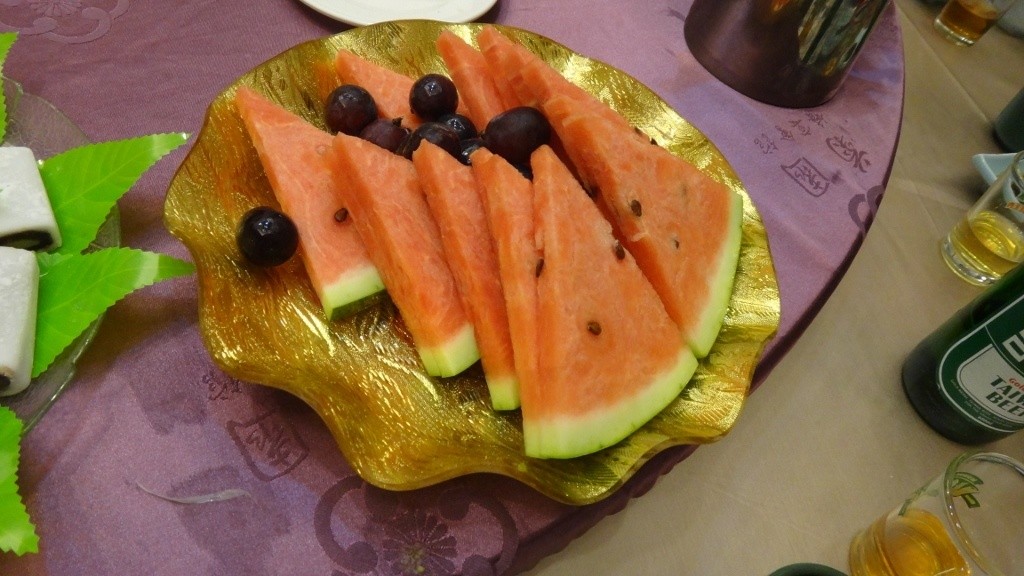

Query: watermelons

(234, 24), (743, 458)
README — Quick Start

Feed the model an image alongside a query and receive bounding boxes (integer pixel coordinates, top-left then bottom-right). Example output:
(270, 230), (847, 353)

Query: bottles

(684, 1), (892, 109)
(902, 261), (1024, 449)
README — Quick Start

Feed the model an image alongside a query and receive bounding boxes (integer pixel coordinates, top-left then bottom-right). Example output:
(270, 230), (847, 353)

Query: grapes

(321, 69), (548, 180)
(236, 205), (298, 268)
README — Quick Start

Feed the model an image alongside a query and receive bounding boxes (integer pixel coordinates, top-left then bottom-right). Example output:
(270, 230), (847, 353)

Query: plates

(0, 74), (126, 438)
(970, 148), (1021, 184)
(160, 20), (780, 507)
(299, 0), (500, 28)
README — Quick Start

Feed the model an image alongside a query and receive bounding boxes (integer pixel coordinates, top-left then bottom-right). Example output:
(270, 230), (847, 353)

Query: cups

(992, 78), (1023, 150)
(851, 452), (1023, 575)
(930, 0), (1015, 50)
(938, 155), (1024, 286)
